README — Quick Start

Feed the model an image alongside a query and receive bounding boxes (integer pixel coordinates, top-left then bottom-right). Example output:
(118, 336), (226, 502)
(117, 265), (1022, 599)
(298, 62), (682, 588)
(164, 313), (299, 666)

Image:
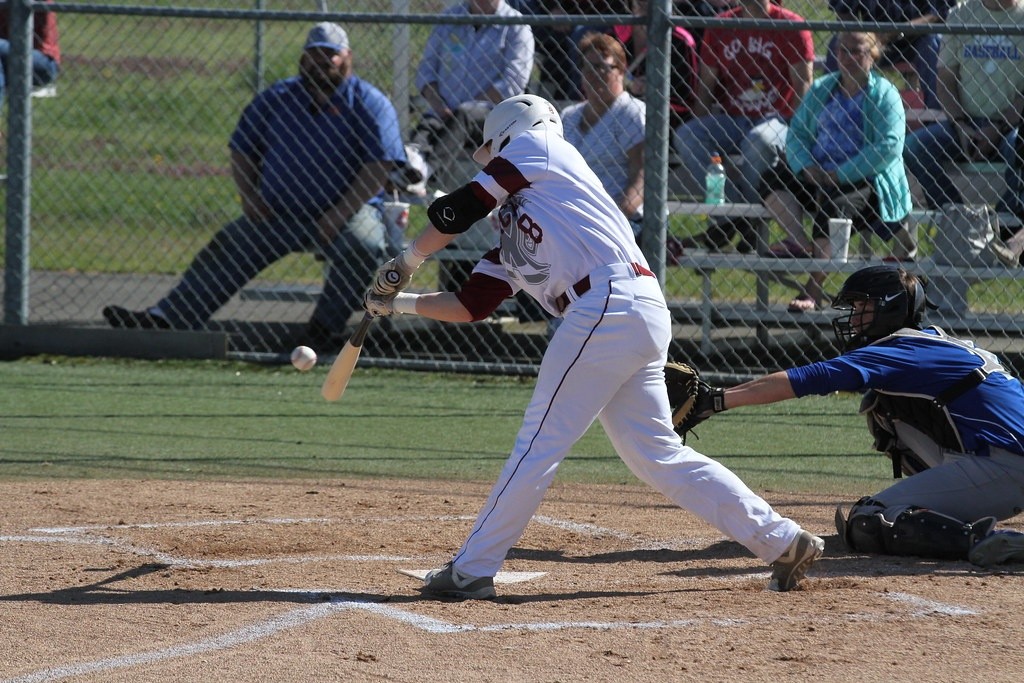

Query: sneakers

(426, 561), (496, 599)
(767, 529), (825, 593)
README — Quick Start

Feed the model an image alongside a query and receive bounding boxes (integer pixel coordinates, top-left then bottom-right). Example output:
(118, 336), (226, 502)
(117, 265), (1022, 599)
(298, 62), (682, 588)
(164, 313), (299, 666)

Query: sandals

(768, 238), (815, 256)
(787, 297), (823, 313)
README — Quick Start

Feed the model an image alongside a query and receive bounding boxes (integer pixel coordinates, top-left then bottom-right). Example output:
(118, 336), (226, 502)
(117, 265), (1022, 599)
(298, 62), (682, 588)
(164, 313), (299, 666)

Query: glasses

(838, 46), (871, 60)
(581, 62), (623, 77)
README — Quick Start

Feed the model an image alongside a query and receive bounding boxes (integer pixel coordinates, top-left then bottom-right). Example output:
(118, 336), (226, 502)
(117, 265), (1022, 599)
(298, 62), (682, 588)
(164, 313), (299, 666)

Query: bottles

(704, 151), (727, 205)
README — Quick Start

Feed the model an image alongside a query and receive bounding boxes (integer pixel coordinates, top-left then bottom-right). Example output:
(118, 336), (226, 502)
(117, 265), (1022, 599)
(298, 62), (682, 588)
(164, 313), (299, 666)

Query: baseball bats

(321, 269), (401, 402)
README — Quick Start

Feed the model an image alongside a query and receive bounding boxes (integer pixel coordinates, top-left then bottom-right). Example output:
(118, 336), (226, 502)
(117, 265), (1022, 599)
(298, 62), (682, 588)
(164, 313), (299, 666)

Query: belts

(555, 262), (656, 312)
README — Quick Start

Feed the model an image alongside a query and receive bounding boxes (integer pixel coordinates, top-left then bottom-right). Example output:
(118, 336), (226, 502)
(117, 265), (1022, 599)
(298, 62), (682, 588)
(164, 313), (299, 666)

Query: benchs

(239, 53), (1024, 367)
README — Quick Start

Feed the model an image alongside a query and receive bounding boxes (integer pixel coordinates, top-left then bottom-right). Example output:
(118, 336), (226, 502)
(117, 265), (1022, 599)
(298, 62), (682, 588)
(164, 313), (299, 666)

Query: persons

(823, 0), (956, 112)
(622, 12), (693, 154)
(361, 95), (827, 600)
(383, 0), (535, 199)
(99, 19), (405, 350)
(901, 0), (1023, 241)
(0, 0), (61, 94)
(754, 30), (911, 316)
(662, 264), (1024, 568)
(559, 31), (645, 238)
(544, 8), (595, 100)
(675, 0), (816, 249)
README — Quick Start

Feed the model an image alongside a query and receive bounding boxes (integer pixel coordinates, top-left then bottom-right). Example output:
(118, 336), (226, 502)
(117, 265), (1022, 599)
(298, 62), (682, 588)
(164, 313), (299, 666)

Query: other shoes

(104, 303), (168, 330)
(969, 527), (1024, 567)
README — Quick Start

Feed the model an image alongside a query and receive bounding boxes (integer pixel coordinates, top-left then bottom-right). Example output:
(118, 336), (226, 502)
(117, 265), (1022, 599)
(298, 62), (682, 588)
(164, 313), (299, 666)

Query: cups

(828, 218), (853, 263)
(383, 201), (410, 248)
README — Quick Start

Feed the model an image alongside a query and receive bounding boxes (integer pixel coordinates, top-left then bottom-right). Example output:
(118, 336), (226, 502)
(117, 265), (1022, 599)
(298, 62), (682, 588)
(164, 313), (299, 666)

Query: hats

(304, 20), (349, 53)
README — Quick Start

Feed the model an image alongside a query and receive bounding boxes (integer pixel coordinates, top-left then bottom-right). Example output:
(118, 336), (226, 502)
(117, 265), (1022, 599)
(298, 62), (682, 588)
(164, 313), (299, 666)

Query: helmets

(833, 266), (927, 354)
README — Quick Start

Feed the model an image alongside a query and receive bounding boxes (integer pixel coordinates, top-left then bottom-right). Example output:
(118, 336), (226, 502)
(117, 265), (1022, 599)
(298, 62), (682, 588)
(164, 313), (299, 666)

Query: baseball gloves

(664, 358), (722, 434)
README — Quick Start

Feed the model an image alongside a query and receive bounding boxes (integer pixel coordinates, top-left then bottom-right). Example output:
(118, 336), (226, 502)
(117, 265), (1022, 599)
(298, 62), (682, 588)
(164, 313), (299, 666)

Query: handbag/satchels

(926, 205), (1001, 264)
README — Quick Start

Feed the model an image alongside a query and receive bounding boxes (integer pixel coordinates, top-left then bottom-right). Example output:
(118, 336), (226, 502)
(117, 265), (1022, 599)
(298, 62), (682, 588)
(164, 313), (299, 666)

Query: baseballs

(291, 345), (317, 372)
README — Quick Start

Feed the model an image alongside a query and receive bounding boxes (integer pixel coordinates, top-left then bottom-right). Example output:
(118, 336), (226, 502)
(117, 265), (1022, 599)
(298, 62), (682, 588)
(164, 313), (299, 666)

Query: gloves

(364, 284), (399, 320)
(373, 250), (413, 301)
